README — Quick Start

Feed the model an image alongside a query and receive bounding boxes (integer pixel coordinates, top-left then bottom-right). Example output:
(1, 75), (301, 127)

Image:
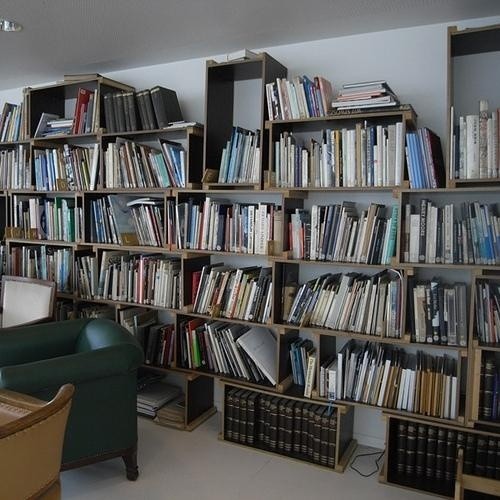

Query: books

(175, 317), (277, 387)
(175, 197), (283, 326)
(388, 419), (500, 500)
(403, 198), (500, 347)
(63, 74), (102, 85)
(102, 136), (187, 187)
(103, 86), (184, 134)
(80, 193), (182, 310)
(135, 379), (185, 429)
(227, 47), (256, 60)
(117, 305), (175, 368)
(263, 75), (400, 122)
(290, 338), (457, 420)
(1, 144), (100, 191)
(219, 100), (498, 190)
(2, 193), (83, 295)
(224, 386), (337, 469)
(52, 300), (116, 322)
(1, 87), (97, 143)
(479, 351), (499, 424)
(287, 202), (403, 339)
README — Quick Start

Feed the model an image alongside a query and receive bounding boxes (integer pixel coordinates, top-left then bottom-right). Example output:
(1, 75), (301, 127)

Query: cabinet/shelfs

(0, 25), (500, 500)
